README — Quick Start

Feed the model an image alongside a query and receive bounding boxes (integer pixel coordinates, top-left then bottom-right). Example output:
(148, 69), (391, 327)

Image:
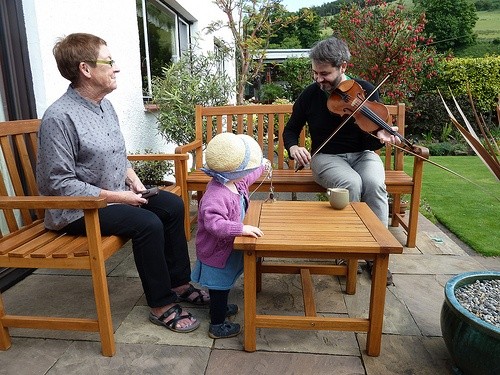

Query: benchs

(0, 119), (191, 357)
(175, 102), (429, 248)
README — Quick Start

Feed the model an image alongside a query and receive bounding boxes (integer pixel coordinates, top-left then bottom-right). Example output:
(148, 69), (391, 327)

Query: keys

(265, 193), (277, 203)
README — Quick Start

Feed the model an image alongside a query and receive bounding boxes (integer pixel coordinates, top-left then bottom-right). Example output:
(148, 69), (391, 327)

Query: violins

(327, 80), (429, 161)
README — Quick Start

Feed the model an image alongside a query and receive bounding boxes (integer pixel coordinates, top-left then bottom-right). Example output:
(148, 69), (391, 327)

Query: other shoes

(367, 261), (393, 286)
(336, 256), (362, 274)
(208, 322), (241, 339)
(226, 304), (238, 318)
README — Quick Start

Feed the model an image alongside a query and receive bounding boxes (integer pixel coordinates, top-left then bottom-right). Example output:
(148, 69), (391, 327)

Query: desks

(234, 200), (404, 357)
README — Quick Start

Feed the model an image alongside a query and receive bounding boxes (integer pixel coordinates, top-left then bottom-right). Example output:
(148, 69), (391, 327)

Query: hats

(201, 133), (263, 185)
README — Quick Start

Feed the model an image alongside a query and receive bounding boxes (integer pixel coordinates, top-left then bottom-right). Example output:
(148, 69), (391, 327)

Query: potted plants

(387, 193), (408, 214)
(128, 150), (176, 188)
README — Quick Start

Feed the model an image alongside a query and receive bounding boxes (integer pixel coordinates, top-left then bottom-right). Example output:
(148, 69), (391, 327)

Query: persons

(191, 132), (271, 338)
(37, 33), (210, 333)
(282, 39), (401, 286)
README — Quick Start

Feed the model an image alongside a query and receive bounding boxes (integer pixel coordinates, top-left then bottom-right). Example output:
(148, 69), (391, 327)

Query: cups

(327, 188), (349, 209)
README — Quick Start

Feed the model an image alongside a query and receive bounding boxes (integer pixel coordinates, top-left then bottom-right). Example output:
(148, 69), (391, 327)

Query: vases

(440, 271), (500, 375)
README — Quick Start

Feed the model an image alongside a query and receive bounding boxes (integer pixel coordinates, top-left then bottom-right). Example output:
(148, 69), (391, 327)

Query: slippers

(175, 284), (211, 304)
(149, 305), (200, 333)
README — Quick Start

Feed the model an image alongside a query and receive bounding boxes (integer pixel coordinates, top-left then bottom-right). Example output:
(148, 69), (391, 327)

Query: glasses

(79, 60), (115, 69)
